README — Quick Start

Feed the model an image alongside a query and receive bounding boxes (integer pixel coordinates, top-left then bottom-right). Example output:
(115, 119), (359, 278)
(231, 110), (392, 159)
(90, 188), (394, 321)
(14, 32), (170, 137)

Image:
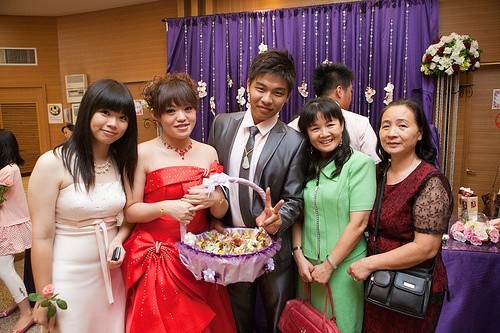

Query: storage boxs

(458, 194), (478, 222)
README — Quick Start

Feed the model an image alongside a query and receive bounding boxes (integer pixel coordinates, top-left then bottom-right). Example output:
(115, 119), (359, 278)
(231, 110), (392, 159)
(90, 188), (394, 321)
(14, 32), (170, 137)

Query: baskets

(178, 176), (282, 286)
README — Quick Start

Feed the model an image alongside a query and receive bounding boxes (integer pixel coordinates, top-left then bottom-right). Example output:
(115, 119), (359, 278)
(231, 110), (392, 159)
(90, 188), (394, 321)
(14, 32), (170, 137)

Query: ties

(238, 126), (260, 228)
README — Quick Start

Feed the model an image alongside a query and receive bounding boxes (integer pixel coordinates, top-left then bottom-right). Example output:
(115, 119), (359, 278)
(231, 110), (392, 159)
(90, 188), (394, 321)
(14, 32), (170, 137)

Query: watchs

(291, 247), (302, 257)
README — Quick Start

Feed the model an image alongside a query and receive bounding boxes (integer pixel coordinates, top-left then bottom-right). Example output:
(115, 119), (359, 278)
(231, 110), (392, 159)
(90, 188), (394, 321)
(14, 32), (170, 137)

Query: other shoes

(9, 318), (36, 333)
(0, 302), (18, 319)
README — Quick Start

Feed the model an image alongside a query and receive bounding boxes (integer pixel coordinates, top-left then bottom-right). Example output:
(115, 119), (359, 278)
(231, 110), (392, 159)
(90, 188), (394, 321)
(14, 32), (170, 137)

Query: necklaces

(94, 157), (111, 174)
(242, 128), (269, 169)
(163, 139), (192, 160)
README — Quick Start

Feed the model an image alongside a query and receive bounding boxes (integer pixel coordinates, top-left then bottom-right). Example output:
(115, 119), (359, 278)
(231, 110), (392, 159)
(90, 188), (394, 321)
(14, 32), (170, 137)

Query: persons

(0, 128), (36, 333)
(208, 48), (303, 333)
(286, 63), (381, 165)
(347, 98), (454, 333)
(124, 71), (236, 333)
(291, 97), (376, 333)
(28, 79), (138, 333)
(61, 123), (74, 140)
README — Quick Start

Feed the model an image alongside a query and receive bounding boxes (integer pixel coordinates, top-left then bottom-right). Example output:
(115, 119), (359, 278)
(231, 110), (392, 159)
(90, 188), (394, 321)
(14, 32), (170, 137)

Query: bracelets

(160, 200), (165, 216)
(326, 255), (337, 269)
(212, 199), (225, 214)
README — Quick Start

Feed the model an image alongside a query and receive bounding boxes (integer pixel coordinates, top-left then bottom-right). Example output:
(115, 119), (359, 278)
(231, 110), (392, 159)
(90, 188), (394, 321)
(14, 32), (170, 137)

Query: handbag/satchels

(364, 268), (434, 320)
(277, 278), (342, 333)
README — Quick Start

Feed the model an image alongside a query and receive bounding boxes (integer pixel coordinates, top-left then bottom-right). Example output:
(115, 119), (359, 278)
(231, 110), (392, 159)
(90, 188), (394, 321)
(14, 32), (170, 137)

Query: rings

(190, 211), (194, 216)
(353, 277), (357, 282)
(273, 208), (276, 214)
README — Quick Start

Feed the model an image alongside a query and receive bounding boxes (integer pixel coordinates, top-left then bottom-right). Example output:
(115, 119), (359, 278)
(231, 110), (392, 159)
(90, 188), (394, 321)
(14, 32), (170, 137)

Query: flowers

(420, 33), (483, 77)
(449, 218), (500, 246)
(28, 284), (67, 333)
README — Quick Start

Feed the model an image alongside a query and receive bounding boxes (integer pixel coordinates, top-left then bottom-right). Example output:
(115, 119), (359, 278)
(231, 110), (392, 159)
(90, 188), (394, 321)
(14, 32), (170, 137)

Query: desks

(434, 238), (500, 333)
